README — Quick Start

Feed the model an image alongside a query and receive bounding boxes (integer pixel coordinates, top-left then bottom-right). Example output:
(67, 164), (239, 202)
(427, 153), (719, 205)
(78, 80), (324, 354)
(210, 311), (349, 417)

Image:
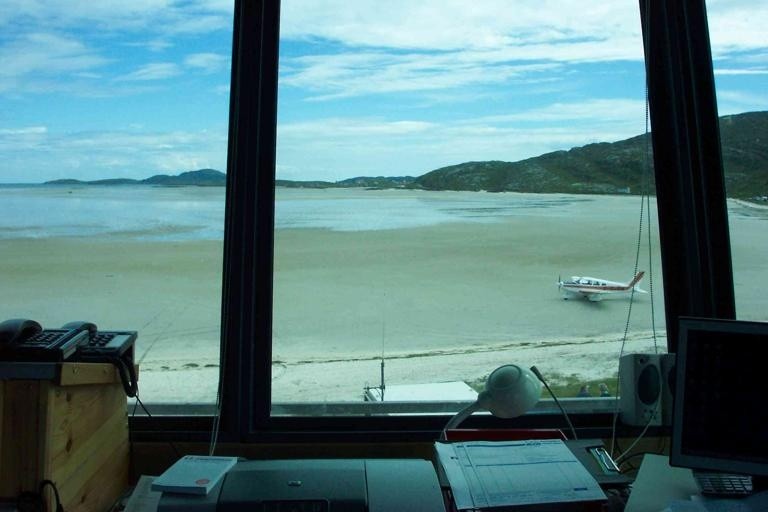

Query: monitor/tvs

(669, 315), (768, 479)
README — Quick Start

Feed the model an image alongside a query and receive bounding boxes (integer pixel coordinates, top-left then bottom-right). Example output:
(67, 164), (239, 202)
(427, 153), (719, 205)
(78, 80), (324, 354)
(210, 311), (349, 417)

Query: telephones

(0, 319), (137, 362)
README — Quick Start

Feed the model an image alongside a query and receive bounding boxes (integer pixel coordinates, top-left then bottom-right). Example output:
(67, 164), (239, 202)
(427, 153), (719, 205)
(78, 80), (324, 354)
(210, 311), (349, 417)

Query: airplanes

(557, 272), (649, 302)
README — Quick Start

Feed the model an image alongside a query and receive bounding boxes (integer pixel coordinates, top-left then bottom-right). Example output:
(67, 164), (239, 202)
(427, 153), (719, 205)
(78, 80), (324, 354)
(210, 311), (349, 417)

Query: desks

(115, 435), (670, 512)
(624, 454), (698, 512)
(0, 362), (139, 512)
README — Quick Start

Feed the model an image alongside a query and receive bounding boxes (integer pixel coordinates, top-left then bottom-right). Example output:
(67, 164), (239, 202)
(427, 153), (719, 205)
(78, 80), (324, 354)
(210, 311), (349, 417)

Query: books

(150, 455), (238, 495)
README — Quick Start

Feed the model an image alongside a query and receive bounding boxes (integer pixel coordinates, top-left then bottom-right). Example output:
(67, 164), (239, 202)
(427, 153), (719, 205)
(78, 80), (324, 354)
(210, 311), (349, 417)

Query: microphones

(531, 364), (579, 440)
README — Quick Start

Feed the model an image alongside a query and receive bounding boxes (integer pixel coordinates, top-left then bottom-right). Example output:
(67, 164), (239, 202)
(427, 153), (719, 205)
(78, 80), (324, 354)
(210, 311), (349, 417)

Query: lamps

(439, 364), (542, 440)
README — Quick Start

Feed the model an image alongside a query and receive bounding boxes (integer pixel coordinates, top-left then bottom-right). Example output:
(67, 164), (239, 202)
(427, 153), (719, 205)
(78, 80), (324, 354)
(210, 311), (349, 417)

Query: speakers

(619, 352), (662, 427)
